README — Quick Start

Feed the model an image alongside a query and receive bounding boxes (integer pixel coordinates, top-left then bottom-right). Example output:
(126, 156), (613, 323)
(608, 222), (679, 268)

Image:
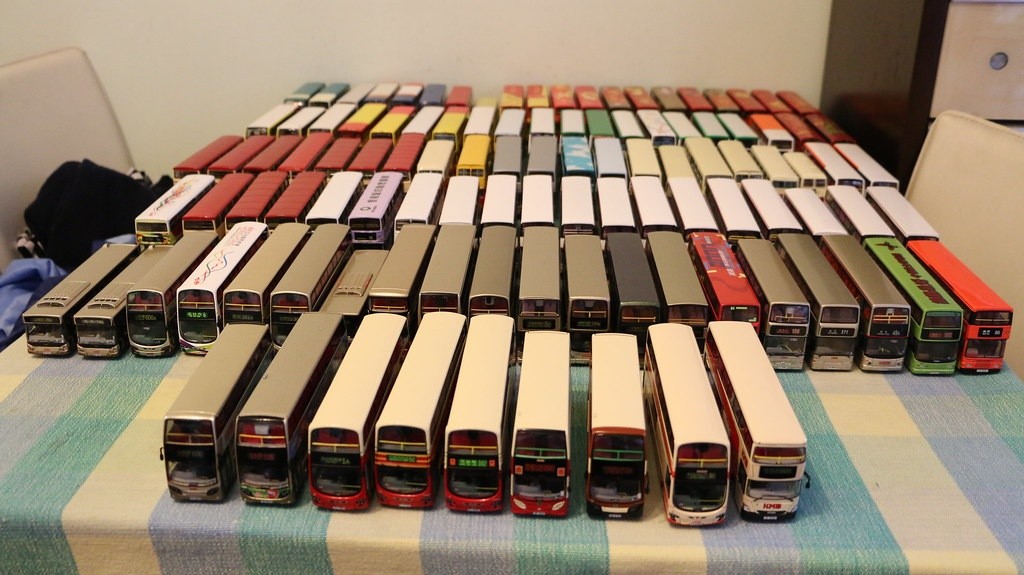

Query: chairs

(0, 47), (140, 278)
(902, 109), (1024, 381)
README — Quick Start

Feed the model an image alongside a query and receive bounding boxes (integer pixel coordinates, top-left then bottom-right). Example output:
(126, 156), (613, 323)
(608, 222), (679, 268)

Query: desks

(0, 332), (1024, 575)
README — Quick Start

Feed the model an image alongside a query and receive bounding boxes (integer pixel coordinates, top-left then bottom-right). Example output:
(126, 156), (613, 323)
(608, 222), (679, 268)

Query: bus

(22, 81), (1014, 528)
